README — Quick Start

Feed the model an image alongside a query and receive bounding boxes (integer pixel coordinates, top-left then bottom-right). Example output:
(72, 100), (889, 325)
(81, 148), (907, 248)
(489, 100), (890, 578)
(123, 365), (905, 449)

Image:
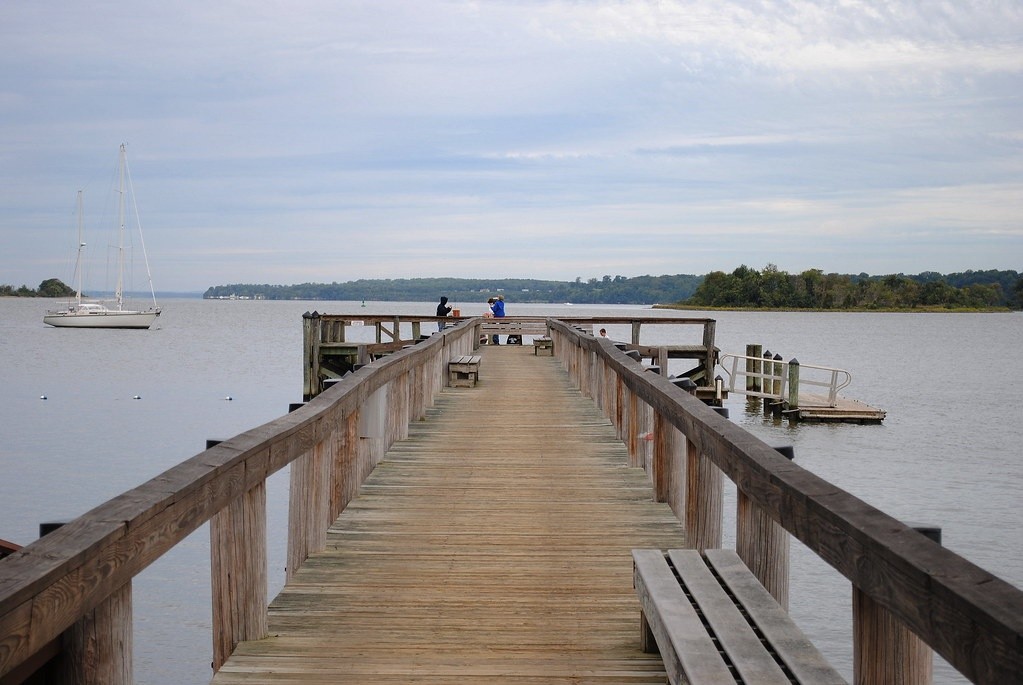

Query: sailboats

(42, 140), (160, 330)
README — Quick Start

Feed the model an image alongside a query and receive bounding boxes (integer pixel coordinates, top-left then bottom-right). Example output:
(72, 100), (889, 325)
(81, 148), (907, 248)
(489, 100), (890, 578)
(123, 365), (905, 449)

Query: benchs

(630, 548), (849, 685)
(532, 336), (553, 356)
(447, 355), (481, 389)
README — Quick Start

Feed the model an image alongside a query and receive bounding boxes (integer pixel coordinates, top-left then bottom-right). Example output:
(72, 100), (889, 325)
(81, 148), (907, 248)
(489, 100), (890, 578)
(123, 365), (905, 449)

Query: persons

(488, 298), (505, 345)
(495, 295), (505, 311)
(437, 297), (452, 333)
(600, 328), (610, 340)
